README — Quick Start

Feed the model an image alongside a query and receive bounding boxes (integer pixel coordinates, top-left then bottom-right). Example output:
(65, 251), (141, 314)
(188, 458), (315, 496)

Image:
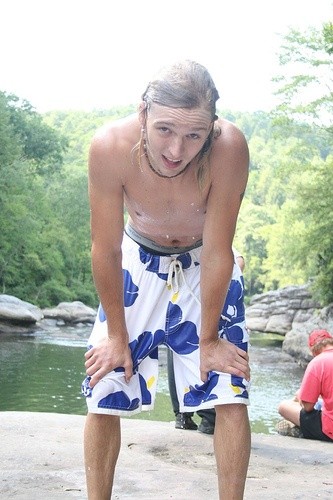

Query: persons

(81, 60), (251, 500)
(275, 329), (333, 442)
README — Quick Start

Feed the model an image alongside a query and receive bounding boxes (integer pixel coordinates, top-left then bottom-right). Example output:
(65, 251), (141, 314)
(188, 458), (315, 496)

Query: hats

(308, 329), (332, 348)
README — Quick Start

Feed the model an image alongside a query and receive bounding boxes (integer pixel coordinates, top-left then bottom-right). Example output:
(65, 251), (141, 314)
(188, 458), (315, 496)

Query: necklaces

(142, 138), (202, 179)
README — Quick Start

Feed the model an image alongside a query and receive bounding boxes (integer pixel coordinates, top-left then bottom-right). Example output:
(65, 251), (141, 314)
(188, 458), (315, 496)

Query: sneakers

(175, 414), (198, 430)
(198, 418), (215, 434)
